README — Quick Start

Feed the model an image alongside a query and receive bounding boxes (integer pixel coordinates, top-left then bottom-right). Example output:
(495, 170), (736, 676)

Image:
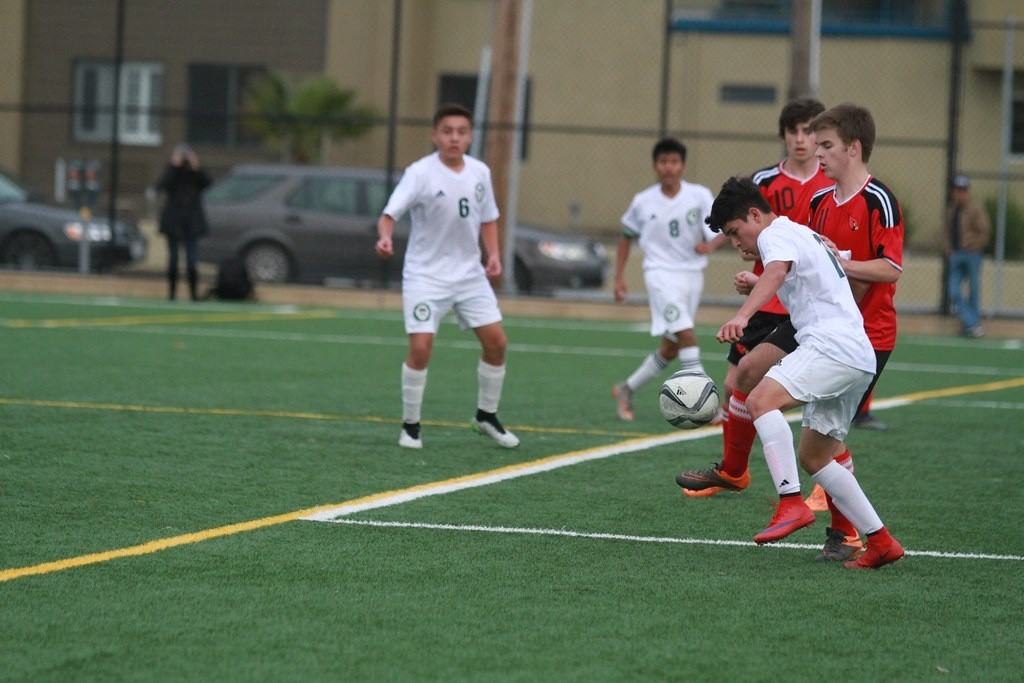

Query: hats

(952, 176), (970, 189)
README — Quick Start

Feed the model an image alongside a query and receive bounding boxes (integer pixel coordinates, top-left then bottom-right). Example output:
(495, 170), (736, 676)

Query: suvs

(194, 164), (614, 296)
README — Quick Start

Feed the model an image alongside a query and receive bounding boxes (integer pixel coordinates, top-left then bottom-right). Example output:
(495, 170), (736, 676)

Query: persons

(676, 103), (905, 562)
(612, 139), (728, 426)
(704, 177), (905, 569)
(375, 104), (521, 449)
(156, 144), (211, 301)
(944, 173), (990, 339)
(686, 97), (836, 511)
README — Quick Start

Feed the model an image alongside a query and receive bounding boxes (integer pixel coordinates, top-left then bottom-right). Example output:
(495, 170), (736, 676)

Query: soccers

(657, 368), (720, 430)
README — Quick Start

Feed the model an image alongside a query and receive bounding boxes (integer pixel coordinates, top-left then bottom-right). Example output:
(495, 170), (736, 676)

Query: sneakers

(815, 528), (863, 564)
(471, 409), (520, 449)
(684, 486), (723, 497)
(613, 384), (634, 422)
(399, 421), (422, 449)
(856, 415), (886, 431)
(753, 497), (816, 546)
(675, 459), (750, 492)
(843, 538), (905, 568)
(804, 483), (829, 511)
(707, 412), (722, 427)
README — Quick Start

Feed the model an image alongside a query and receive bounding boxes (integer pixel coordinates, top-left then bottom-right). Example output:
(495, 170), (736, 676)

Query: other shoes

(963, 325), (985, 337)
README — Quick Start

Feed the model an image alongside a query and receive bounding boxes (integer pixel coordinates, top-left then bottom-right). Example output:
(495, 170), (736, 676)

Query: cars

(1, 168), (142, 273)
